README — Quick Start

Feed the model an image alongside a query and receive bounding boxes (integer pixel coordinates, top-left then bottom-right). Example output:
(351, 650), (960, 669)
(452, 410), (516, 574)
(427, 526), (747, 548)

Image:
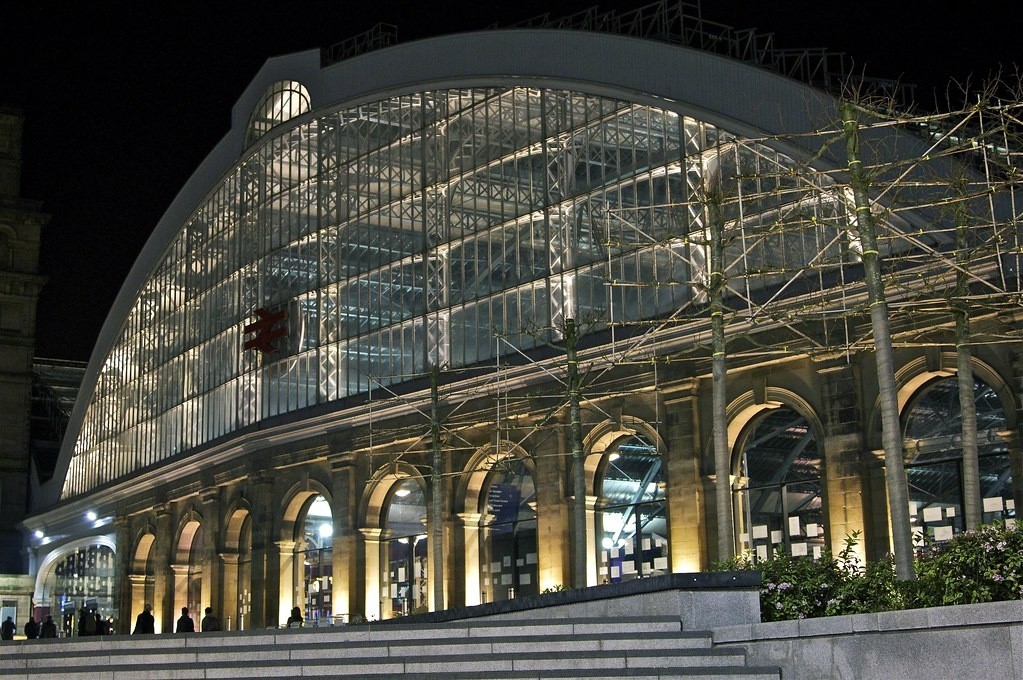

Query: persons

(202, 607), (221, 631)
(176, 607), (194, 632)
(133, 604), (154, 634)
(39, 616), (59, 638)
(94, 614), (109, 634)
(288, 607), (303, 626)
(25, 617), (39, 639)
(1, 616), (16, 640)
(78, 608), (94, 636)
